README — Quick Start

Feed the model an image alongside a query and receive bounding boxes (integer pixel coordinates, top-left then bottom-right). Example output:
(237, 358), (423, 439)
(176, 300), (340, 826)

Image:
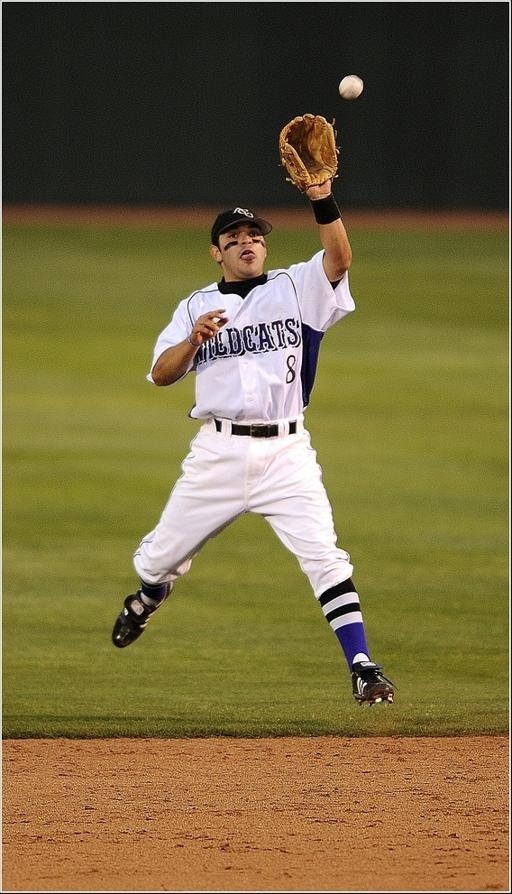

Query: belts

(216, 418), (296, 437)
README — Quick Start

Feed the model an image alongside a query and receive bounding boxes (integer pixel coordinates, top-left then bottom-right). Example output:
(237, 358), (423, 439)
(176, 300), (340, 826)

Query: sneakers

(110, 581), (174, 648)
(352, 661), (400, 705)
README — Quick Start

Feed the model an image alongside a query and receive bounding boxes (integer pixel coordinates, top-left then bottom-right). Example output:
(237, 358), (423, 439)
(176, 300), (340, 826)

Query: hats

(210, 206), (272, 243)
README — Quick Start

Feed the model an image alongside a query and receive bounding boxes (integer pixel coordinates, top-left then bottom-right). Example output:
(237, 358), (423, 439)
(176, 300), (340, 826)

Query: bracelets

(187, 336), (200, 349)
(309, 192), (342, 225)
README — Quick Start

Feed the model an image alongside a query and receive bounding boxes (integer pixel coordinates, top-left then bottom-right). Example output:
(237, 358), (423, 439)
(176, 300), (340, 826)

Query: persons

(111, 113), (396, 705)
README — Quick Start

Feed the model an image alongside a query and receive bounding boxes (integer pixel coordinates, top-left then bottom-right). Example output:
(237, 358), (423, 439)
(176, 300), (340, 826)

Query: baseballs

(339, 75), (364, 100)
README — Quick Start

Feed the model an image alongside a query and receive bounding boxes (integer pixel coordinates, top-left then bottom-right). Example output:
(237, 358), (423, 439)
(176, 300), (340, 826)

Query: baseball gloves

(278, 113), (341, 196)
